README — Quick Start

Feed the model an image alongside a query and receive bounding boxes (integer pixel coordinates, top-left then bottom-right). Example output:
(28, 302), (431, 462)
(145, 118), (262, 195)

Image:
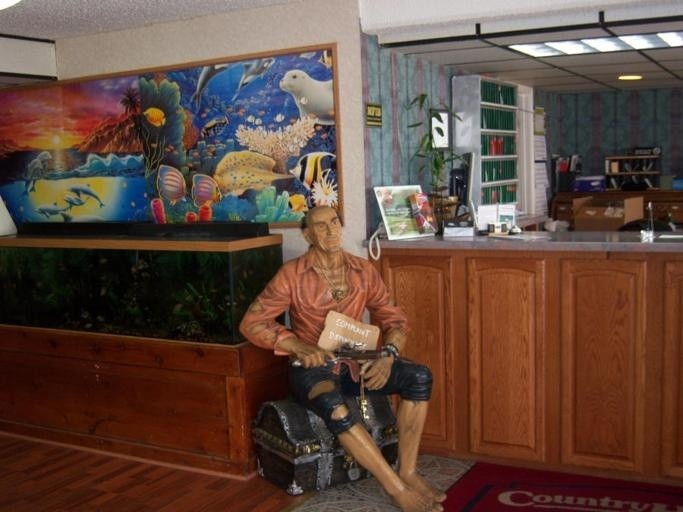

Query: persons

(237, 203), (456, 512)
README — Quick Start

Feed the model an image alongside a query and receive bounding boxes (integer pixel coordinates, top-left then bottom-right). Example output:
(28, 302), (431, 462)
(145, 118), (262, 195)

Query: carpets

(439, 461), (681, 511)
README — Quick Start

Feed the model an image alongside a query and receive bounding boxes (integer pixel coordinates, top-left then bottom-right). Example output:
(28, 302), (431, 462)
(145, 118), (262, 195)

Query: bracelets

(380, 343), (399, 361)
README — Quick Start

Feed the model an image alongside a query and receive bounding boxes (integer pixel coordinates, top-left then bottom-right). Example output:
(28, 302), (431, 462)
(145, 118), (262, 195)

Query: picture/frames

(429, 108), (452, 151)
(0, 40), (346, 230)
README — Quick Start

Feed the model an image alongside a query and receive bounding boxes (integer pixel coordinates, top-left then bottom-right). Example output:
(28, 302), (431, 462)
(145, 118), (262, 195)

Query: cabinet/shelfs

(603, 154), (661, 191)
(450, 73), (535, 231)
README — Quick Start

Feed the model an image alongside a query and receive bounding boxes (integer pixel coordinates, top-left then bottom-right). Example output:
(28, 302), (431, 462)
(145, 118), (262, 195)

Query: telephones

(377, 221), (387, 239)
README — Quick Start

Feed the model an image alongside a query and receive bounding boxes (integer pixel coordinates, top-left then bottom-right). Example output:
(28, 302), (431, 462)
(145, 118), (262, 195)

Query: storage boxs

(571, 196), (644, 231)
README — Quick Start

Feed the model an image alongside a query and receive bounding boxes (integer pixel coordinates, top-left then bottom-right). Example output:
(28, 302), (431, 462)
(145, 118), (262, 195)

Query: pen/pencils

(648, 201), (655, 230)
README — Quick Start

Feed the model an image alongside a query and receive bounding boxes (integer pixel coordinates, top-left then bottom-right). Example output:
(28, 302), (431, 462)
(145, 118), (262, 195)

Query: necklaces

(316, 254), (345, 302)
